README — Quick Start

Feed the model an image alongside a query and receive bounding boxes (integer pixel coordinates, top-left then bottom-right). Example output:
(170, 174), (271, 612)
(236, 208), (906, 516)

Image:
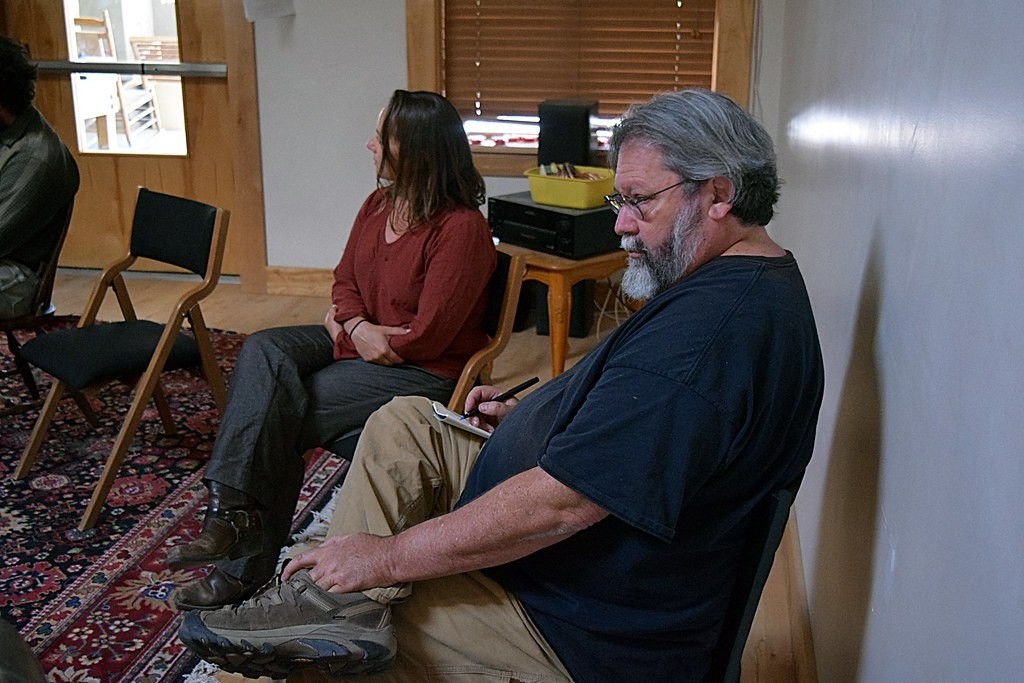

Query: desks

(74, 55), (122, 152)
(486, 240), (649, 381)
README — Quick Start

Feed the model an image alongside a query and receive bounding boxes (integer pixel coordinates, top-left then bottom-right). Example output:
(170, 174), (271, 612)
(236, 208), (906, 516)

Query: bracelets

(349, 318), (368, 339)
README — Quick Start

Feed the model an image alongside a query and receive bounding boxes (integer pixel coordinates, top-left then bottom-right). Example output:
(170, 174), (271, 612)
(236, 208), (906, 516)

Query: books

(431, 401), (491, 439)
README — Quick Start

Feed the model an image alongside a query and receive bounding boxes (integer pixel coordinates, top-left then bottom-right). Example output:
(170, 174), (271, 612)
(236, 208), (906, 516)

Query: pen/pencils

(457, 375), (542, 421)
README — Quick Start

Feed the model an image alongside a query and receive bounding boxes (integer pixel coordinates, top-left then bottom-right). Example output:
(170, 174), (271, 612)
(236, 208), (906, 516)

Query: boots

(165, 480), (258, 564)
(174, 568), (275, 611)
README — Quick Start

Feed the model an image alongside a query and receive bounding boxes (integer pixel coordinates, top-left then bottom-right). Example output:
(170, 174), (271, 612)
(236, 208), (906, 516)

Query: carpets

(0, 315), (354, 683)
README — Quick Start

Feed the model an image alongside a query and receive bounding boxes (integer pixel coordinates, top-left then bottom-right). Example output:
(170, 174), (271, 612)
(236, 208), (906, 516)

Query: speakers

(538, 99), (598, 166)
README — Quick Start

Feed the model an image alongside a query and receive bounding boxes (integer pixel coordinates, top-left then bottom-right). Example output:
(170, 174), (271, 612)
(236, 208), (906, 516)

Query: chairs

(1, 192), (101, 429)
(321, 243), (527, 462)
(714, 476), (797, 683)
(74, 9), (187, 154)
(13, 186), (231, 534)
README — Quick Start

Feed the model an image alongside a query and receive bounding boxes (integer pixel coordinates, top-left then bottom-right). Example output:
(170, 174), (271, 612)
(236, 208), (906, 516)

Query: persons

(179, 90), (826, 682)
(164, 95), (499, 605)
(0, 37), (80, 319)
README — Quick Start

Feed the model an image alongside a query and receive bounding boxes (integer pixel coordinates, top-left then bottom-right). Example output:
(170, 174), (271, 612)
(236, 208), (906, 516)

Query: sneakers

(176, 568), (399, 680)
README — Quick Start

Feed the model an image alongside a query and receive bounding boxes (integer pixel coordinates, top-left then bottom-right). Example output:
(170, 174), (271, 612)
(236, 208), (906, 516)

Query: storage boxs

(523, 164), (616, 210)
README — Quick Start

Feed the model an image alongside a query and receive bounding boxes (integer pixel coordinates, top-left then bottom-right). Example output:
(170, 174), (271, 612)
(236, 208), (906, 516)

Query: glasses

(603, 179), (691, 221)
